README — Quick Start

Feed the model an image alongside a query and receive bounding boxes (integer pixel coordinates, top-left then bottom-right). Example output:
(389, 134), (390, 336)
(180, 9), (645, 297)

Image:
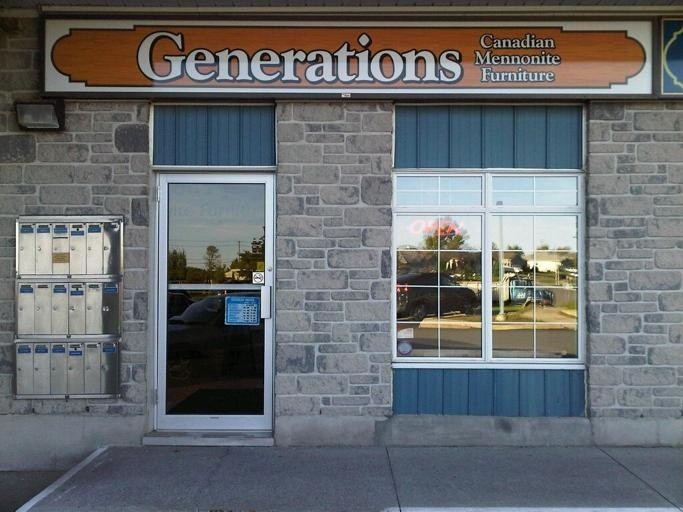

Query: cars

(167, 281), (264, 386)
(397, 272), (479, 321)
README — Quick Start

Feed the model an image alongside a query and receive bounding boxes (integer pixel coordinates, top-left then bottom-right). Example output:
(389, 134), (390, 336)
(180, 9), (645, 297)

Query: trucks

(459, 273), (554, 305)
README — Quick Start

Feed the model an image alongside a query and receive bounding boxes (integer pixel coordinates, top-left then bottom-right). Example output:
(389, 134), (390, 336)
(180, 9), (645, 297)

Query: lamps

(15, 99), (64, 132)
